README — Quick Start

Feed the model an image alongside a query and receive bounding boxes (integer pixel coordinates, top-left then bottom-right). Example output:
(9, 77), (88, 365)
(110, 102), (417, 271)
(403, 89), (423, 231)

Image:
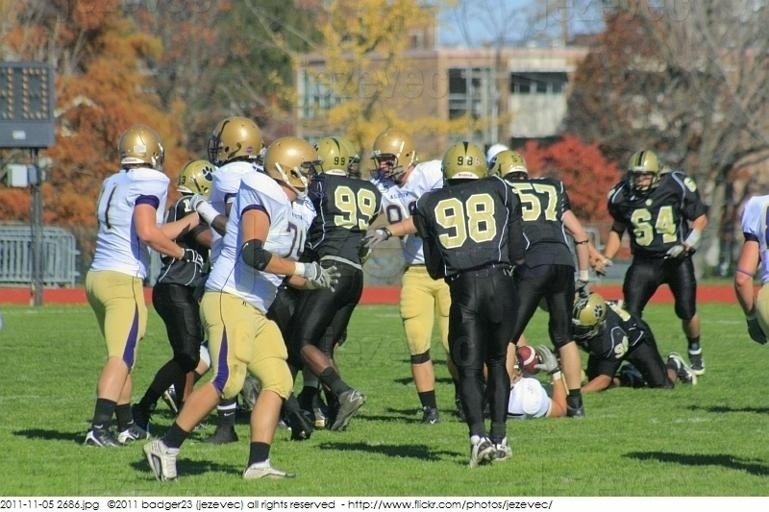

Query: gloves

(746, 303), (769, 345)
(532, 344), (561, 375)
(596, 256), (614, 277)
(304, 261), (341, 293)
(180, 248), (203, 269)
(361, 227), (392, 249)
(664, 242), (689, 260)
(577, 279), (590, 298)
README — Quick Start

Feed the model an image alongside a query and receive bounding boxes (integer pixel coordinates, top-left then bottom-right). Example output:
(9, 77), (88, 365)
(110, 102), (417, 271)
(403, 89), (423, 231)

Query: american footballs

(516, 346), (542, 374)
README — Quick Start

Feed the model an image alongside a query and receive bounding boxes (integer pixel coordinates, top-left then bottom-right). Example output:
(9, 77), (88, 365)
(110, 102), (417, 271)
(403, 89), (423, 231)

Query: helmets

(207, 116), (264, 167)
(483, 342), (524, 386)
(626, 149), (659, 198)
(119, 125), (165, 171)
(264, 136), (360, 203)
(572, 290), (607, 345)
(369, 128), (419, 189)
(441, 141), (529, 187)
(175, 160), (219, 199)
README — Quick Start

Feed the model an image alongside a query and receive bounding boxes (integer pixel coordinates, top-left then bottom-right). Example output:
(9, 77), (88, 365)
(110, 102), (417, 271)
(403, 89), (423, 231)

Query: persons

(734, 195), (769, 345)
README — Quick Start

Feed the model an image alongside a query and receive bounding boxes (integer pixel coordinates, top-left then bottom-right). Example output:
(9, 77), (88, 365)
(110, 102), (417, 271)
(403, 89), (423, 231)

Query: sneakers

(241, 457), (296, 479)
(162, 384), (178, 415)
(687, 347), (704, 376)
(618, 363), (647, 389)
(466, 434), (512, 468)
(278, 387), (367, 441)
(667, 352), (697, 385)
(143, 439), (180, 482)
(567, 403), (584, 416)
(202, 427), (238, 444)
(82, 403), (151, 447)
(420, 405), (440, 424)
